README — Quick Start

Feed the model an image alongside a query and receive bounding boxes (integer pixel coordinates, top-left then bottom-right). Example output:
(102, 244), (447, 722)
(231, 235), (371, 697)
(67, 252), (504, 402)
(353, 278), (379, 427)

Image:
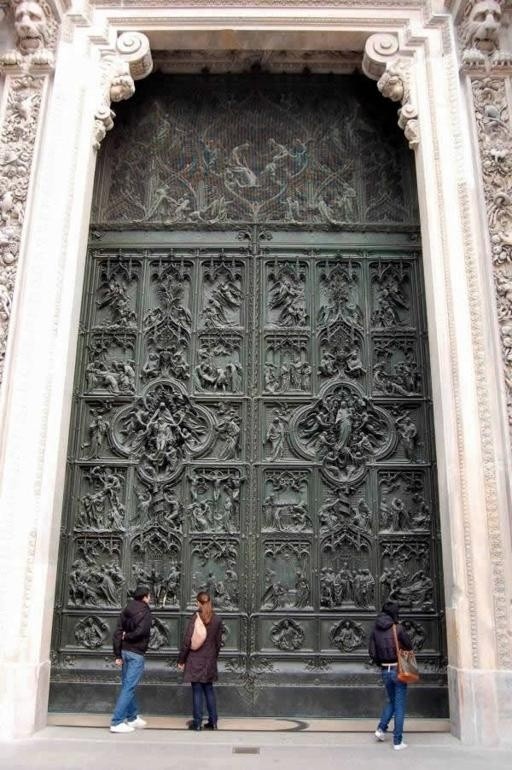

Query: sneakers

(374, 728), (385, 741)
(125, 715), (147, 727)
(393, 740), (408, 750)
(109, 722), (136, 733)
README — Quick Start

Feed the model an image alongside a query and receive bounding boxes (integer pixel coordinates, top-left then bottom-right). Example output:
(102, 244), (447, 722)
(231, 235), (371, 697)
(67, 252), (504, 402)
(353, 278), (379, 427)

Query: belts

(380, 665), (397, 669)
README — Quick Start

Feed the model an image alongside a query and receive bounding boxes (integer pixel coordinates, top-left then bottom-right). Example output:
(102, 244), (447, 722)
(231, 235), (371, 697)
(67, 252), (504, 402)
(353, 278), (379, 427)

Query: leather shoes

(187, 719), (217, 731)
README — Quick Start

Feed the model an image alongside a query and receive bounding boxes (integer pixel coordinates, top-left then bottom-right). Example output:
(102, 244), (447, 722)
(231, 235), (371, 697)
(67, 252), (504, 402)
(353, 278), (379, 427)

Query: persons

(109, 586), (153, 735)
(367, 600), (420, 752)
(177, 591), (225, 732)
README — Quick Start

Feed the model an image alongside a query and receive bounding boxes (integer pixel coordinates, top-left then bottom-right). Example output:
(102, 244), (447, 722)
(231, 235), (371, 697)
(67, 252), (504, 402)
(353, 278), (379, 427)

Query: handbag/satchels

(189, 612), (209, 652)
(392, 623), (420, 685)
